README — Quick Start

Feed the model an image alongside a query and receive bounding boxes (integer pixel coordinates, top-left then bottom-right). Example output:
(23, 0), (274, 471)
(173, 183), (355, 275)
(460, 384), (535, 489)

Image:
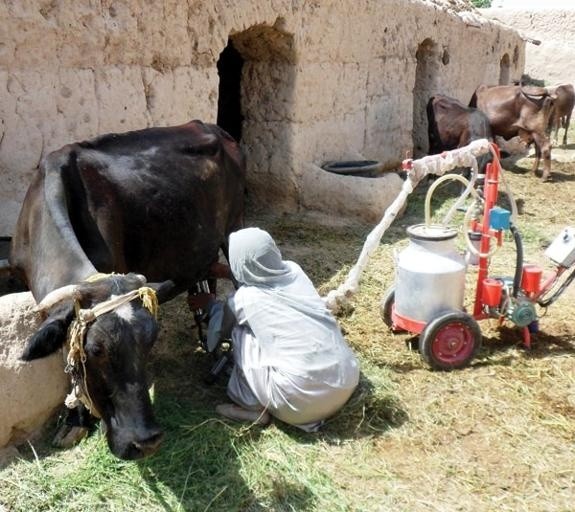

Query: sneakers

(215, 404), (269, 425)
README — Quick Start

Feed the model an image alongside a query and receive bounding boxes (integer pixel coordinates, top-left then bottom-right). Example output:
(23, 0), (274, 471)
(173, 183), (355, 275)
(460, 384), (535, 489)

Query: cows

(545, 82), (575, 148)
(8, 118), (249, 463)
(425, 94), (491, 185)
(466, 85), (556, 183)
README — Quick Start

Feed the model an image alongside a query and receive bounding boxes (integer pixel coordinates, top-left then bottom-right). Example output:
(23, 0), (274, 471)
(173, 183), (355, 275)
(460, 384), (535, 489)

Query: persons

(188, 226), (361, 434)
